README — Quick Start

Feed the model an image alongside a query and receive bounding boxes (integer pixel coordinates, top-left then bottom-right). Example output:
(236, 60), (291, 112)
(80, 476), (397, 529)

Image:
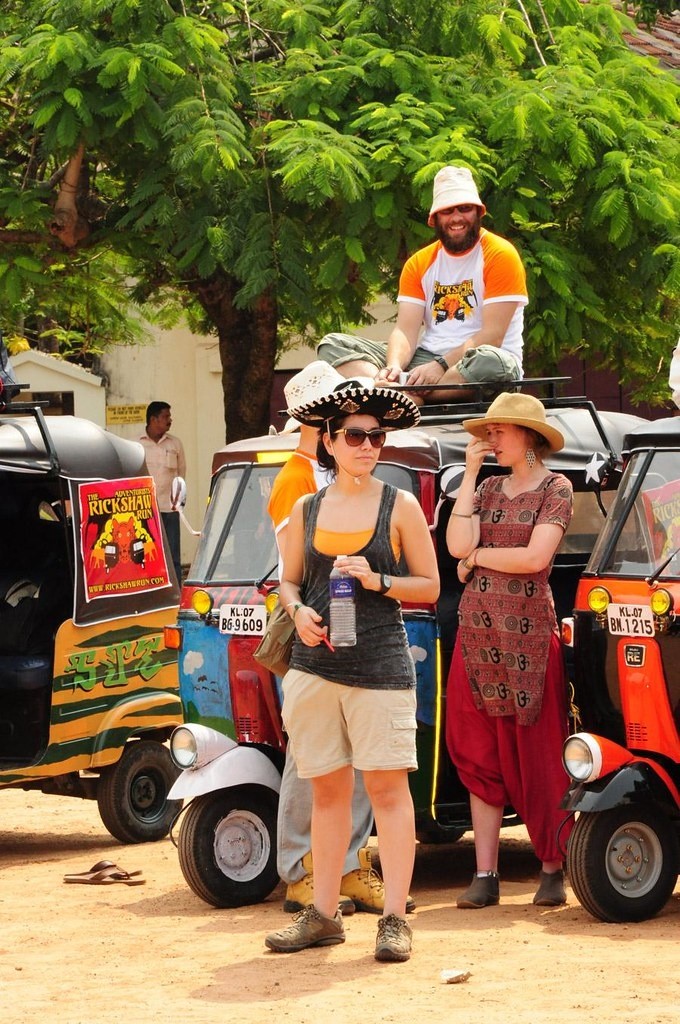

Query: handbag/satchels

(252, 597), (296, 678)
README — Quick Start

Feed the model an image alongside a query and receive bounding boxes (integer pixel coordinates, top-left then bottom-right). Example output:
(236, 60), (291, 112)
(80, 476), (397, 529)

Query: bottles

(330, 556), (357, 646)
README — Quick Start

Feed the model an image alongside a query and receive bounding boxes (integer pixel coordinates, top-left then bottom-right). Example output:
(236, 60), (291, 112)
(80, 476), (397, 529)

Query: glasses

(439, 204), (473, 215)
(332, 428), (387, 449)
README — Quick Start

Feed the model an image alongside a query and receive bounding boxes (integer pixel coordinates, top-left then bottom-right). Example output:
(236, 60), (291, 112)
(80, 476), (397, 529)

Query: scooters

(167, 376), (679, 909)
(557, 414), (679, 923)
(0, 382), (187, 846)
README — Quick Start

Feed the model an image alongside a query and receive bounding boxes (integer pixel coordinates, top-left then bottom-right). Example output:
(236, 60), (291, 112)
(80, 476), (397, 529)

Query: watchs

(377, 572), (392, 596)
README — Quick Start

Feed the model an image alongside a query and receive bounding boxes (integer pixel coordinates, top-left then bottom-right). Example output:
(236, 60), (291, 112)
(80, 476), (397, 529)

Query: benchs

(0, 518), (72, 756)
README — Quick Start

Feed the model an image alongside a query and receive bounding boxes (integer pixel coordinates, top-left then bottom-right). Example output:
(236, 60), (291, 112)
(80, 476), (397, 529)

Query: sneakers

(341, 848), (417, 914)
(374, 913), (413, 961)
(264, 904), (346, 953)
(283, 852), (356, 914)
(533, 870), (567, 905)
(456, 874), (501, 908)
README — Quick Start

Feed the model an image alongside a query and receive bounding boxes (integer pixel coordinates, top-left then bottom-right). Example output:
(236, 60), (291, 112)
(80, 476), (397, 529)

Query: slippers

(63, 860), (146, 886)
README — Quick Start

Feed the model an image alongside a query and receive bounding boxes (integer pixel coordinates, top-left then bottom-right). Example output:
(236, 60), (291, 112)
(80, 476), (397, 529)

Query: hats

(278, 360), (376, 439)
(287, 380), (421, 432)
(463, 392), (564, 453)
(428, 166), (486, 228)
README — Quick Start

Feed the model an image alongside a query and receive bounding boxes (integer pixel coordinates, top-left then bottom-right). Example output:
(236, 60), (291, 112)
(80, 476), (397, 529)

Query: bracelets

(461, 556), (473, 570)
(292, 602), (305, 621)
(434, 356), (449, 372)
(451, 511), (472, 519)
(474, 546), (483, 566)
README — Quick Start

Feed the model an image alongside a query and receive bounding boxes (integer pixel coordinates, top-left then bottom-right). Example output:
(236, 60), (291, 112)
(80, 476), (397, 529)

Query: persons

(444, 391), (576, 910)
(267, 360), (417, 917)
(137, 400), (187, 591)
(260, 380), (442, 964)
(315, 166), (530, 407)
